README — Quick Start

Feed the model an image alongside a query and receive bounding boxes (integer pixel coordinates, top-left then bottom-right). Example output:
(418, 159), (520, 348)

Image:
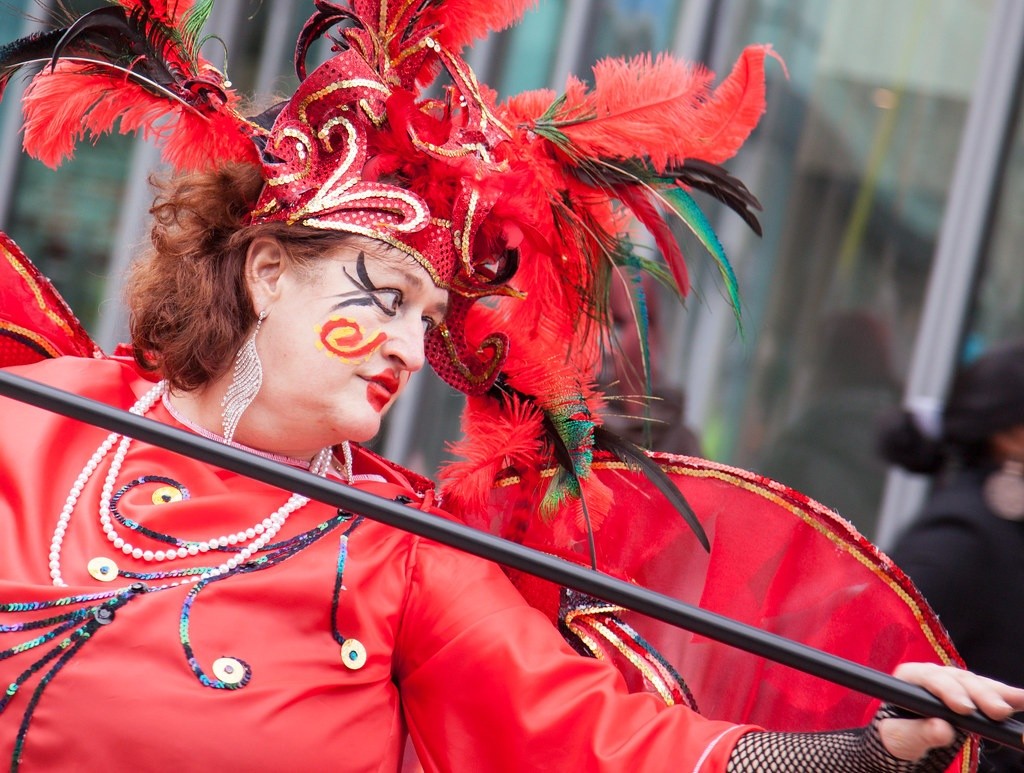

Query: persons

(592, 266), (699, 458)
(871, 348), (1024, 773)
(0, 48), (1024, 773)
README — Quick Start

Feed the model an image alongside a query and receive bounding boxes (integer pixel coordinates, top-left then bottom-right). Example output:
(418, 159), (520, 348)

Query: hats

(941, 347), (1024, 436)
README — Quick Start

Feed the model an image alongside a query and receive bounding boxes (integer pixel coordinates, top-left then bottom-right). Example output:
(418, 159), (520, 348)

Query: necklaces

(49, 379), (333, 598)
